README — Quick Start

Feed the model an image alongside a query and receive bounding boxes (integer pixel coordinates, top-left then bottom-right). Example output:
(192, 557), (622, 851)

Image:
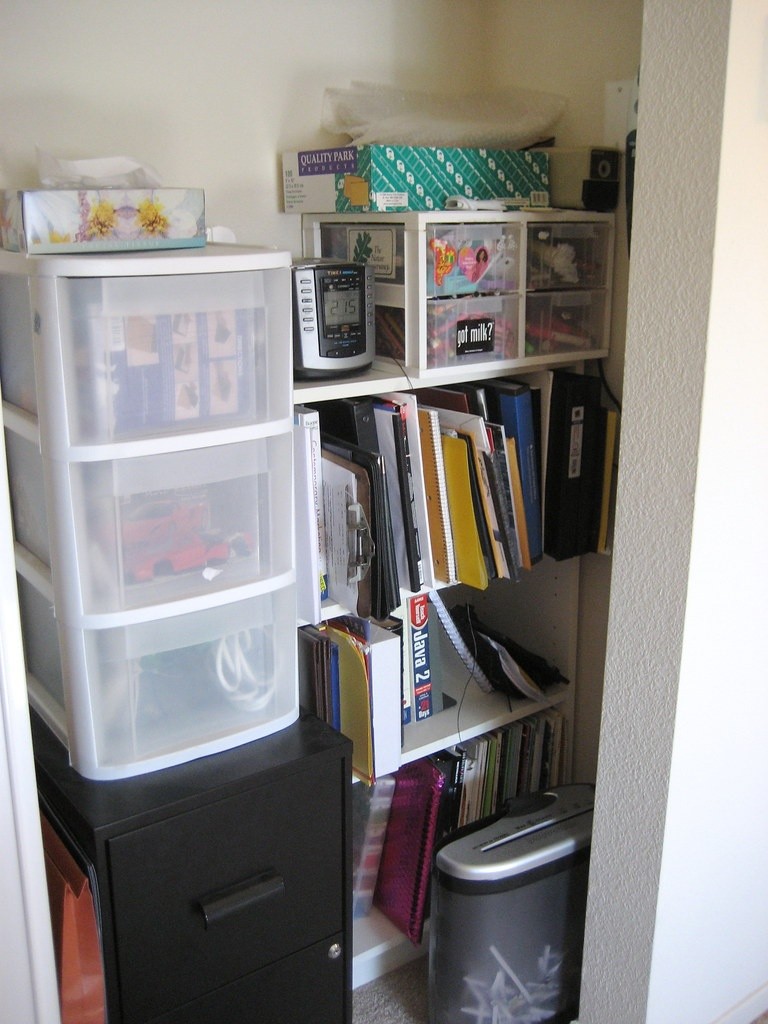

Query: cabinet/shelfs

(0, 241), (300, 781)
(287, 203), (619, 994)
(29, 705), (355, 1024)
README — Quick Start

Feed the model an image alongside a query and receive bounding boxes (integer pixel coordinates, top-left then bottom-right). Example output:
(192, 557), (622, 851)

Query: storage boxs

(526, 222), (613, 291)
(320, 222), (523, 301)
(374, 293), (519, 372)
(524, 292), (606, 358)
(283, 144), (549, 213)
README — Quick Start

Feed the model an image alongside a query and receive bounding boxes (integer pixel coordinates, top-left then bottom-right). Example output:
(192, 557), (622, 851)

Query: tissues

(0, 142), (207, 254)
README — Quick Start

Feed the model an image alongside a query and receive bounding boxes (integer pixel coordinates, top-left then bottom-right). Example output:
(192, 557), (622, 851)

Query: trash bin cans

(428, 778), (599, 1023)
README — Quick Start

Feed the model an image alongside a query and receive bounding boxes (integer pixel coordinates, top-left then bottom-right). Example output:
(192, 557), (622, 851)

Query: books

(296, 369), (622, 945)
